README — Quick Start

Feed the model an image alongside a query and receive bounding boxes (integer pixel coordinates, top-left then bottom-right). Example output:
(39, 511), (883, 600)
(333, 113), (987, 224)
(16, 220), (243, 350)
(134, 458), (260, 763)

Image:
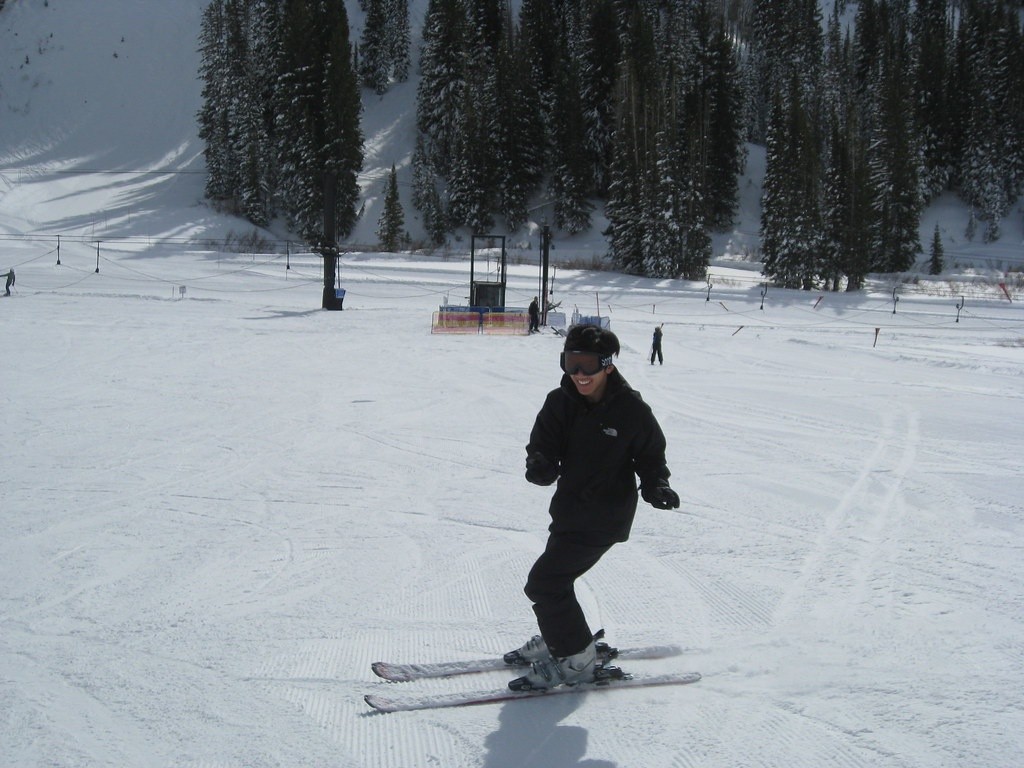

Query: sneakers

(509, 629), (605, 691)
(503, 635), (552, 665)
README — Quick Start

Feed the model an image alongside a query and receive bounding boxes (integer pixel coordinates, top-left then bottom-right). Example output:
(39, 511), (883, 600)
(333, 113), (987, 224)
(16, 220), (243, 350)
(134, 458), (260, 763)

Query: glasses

(559, 351), (612, 375)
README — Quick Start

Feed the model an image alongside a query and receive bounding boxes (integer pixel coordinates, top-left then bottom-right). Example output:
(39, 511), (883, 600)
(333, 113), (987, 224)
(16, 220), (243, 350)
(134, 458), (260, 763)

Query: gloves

(637, 473), (680, 510)
(524, 443), (555, 482)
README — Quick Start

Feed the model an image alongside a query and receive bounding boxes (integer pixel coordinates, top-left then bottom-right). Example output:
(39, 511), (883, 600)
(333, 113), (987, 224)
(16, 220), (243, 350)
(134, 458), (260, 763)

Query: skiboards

(364, 641), (703, 711)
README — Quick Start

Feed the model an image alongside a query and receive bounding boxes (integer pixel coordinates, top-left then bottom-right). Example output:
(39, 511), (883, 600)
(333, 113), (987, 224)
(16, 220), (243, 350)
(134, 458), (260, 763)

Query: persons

(651, 328), (663, 365)
(528, 296), (541, 334)
(503, 323), (682, 692)
(0, 268), (15, 296)
